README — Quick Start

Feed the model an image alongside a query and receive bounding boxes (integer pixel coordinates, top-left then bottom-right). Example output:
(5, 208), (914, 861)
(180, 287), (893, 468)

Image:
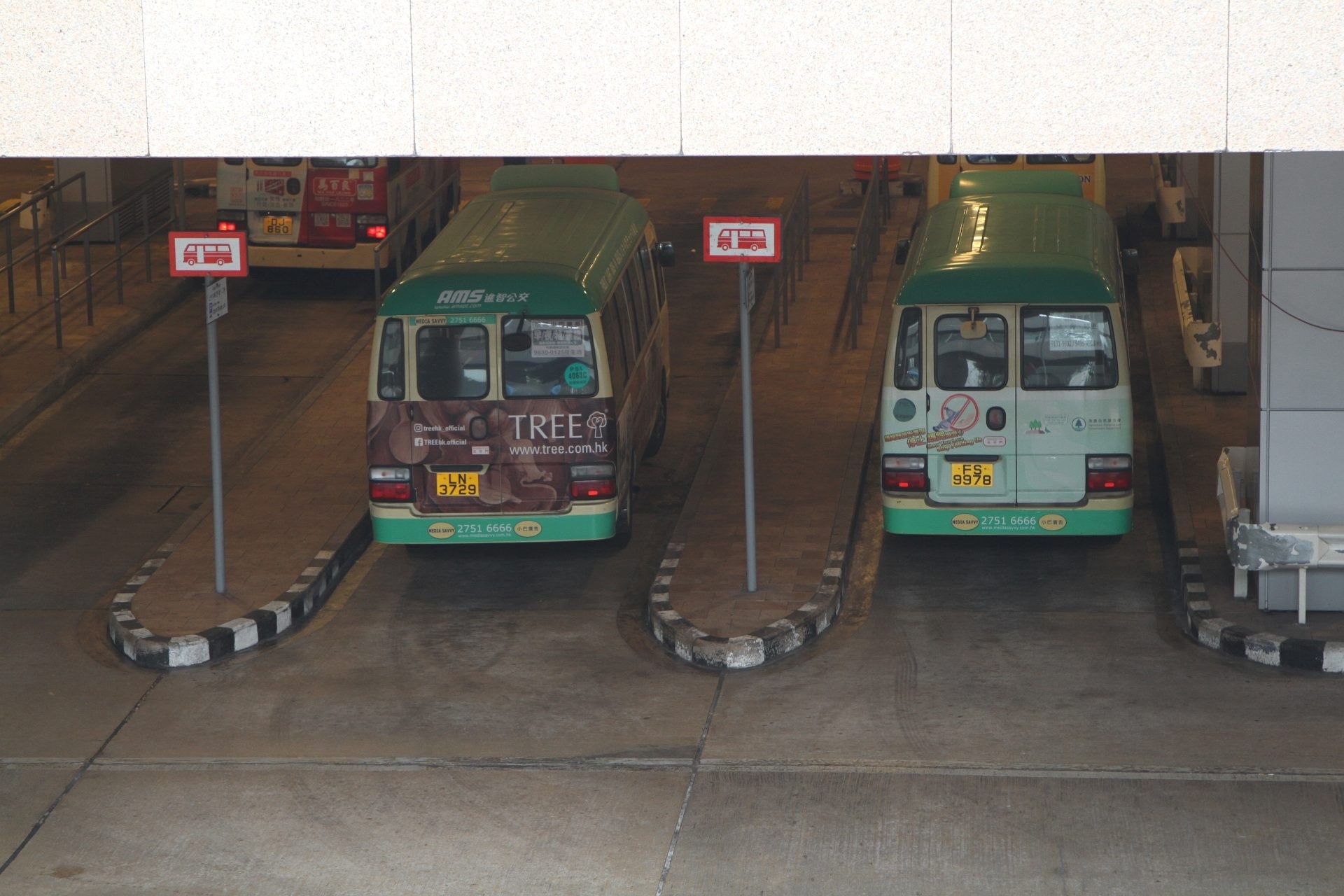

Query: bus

(365, 163), (678, 549)
(929, 154), (1108, 208)
(217, 156), (461, 281)
(878, 170), (1135, 536)
